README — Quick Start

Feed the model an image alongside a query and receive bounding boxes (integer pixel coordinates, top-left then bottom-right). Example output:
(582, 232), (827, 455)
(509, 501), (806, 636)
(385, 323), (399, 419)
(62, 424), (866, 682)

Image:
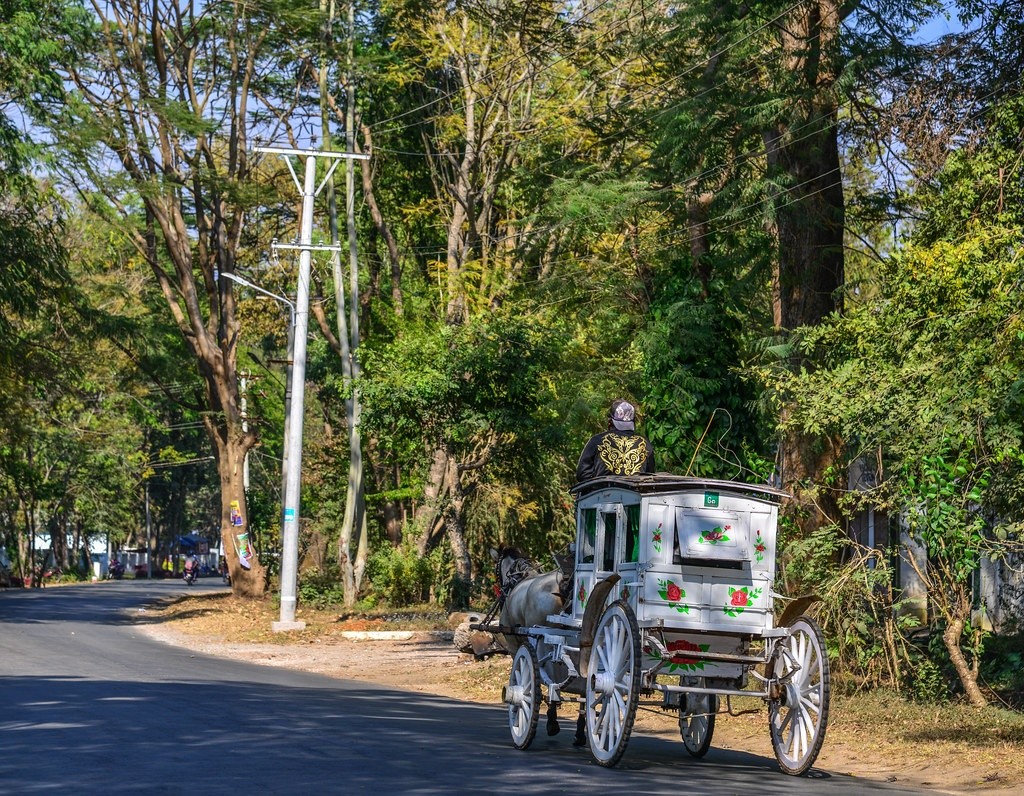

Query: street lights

(222, 271), (307, 625)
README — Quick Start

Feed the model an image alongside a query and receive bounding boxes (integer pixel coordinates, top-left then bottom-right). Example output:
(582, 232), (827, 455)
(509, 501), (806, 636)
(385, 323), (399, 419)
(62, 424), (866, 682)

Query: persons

(573, 400), (656, 562)
(183, 550), (200, 582)
(220, 555), (227, 582)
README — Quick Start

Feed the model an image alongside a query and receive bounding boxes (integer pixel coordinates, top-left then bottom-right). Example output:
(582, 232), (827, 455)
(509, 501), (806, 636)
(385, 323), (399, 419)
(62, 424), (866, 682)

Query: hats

(606, 400), (635, 431)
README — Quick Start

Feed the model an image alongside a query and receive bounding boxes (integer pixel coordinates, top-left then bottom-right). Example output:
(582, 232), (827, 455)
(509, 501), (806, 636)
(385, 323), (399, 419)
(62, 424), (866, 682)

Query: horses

(488, 541), (587, 747)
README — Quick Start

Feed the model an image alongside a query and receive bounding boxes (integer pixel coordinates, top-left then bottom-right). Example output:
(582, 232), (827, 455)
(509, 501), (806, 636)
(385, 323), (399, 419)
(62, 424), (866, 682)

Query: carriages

(470, 473), (830, 777)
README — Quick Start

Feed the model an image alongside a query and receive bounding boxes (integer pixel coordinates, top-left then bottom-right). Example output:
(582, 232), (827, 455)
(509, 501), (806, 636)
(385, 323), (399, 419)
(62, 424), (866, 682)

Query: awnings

(176, 534), (211, 546)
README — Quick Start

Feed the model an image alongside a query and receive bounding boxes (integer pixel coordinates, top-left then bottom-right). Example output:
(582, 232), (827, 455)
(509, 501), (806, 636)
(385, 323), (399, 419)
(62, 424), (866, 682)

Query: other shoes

(184, 577), (186, 579)
(193, 579), (197, 581)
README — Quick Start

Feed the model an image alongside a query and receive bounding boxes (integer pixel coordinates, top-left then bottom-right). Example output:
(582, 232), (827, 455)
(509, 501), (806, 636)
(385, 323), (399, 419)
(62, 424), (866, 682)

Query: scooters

(106, 559), (125, 579)
(185, 570), (194, 585)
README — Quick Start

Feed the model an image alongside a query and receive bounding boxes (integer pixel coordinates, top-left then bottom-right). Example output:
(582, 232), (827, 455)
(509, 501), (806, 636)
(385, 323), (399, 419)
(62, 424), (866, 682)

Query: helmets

(186, 550), (192, 556)
